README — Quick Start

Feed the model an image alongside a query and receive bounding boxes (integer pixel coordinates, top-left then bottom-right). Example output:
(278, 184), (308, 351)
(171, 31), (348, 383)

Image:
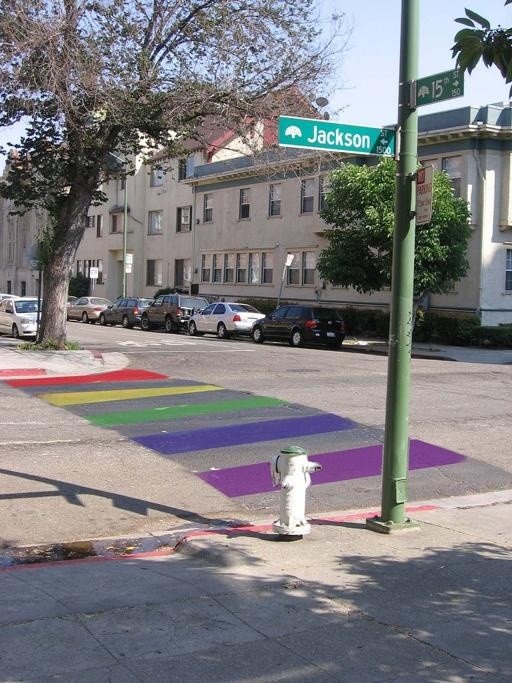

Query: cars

(100, 298), (156, 329)
(188, 302), (266, 338)
(0, 296), (44, 340)
(66, 296), (112, 324)
(248, 304), (346, 351)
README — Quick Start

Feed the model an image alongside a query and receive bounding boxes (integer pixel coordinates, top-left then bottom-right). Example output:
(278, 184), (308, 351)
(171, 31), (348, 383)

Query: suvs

(139, 292), (209, 335)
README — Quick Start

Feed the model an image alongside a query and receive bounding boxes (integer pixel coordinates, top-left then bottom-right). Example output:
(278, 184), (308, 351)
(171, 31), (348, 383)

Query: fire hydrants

(269, 444), (321, 539)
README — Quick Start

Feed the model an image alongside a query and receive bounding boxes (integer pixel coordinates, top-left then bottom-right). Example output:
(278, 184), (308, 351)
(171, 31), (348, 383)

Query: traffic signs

(407, 69), (468, 110)
(278, 113), (403, 163)
